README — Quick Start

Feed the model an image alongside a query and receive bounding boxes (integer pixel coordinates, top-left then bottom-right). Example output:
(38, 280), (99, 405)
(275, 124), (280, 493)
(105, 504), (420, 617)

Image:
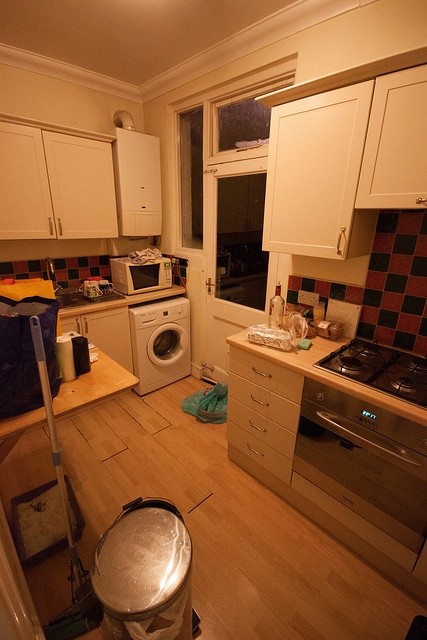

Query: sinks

(53, 291), (96, 309)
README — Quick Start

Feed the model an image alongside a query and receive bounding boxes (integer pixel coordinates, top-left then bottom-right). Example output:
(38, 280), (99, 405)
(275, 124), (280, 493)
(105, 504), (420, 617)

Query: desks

(0, 339), (141, 448)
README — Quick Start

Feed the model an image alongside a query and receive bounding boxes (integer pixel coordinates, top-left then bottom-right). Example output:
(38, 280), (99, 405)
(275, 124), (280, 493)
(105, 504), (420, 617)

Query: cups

(79, 280), (89, 296)
(85, 285), (103, 298)
(99, 280), (114, 295)
(89, 280), (98, 285)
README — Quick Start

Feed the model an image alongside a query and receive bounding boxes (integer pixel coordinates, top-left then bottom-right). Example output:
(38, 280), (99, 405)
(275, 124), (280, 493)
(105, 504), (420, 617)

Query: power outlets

(171, 259), (179, 264)
(297, 290), (320, 308)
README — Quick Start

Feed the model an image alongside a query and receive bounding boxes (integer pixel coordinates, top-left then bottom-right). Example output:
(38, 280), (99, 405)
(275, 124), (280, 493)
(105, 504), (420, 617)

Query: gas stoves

(312, 336), (427, 412)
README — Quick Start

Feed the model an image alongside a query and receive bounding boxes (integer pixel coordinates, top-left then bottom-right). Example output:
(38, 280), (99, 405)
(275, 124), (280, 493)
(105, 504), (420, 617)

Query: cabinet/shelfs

(261, 74), (381, 263)
(354, 60), (427, 210)
(0, 121), (120, 241)
(60, 305), (133, 374)
(217, 173), (267, 235)
(225, 335), (307, 486)
(109, 127), (163, 238)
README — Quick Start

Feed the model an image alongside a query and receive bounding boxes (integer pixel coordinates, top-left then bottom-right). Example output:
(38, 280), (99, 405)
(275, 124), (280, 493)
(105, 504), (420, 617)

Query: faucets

(45, 256), (63, 294)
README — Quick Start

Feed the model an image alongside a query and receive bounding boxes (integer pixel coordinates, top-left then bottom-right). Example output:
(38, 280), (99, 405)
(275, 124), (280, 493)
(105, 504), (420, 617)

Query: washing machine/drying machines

(129, 296), (191, 397)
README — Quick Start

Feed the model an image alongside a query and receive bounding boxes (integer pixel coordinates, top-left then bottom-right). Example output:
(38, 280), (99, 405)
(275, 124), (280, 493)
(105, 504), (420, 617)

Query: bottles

(268, 280), (284, 329)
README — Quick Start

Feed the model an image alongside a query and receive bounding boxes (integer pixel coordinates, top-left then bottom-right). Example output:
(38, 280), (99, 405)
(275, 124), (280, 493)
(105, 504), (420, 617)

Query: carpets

(404, 614), (427, 639)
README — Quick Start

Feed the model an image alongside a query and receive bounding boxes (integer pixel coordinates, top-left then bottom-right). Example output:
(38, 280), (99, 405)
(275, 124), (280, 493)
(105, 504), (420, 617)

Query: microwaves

(110, 256), (173, 296)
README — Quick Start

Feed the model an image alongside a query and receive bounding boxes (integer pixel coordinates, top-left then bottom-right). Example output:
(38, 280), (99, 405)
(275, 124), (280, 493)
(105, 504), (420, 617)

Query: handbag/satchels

(0, 295), (62, 418)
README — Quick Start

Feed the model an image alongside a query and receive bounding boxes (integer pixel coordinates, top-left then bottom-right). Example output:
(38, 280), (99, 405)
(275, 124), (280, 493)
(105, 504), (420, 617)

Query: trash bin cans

(88, 496), (193, 640)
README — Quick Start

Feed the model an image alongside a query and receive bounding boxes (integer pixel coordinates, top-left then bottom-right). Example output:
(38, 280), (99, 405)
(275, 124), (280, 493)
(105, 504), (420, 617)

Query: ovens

(289, 371), (427, 573)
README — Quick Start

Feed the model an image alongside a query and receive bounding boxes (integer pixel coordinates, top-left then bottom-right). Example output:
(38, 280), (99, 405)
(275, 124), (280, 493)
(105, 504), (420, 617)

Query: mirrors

(215, 171), (270, 312)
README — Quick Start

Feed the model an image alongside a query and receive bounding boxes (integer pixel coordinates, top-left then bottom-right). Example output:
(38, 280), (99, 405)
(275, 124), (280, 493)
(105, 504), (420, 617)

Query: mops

(29, 315), (103, 640)
